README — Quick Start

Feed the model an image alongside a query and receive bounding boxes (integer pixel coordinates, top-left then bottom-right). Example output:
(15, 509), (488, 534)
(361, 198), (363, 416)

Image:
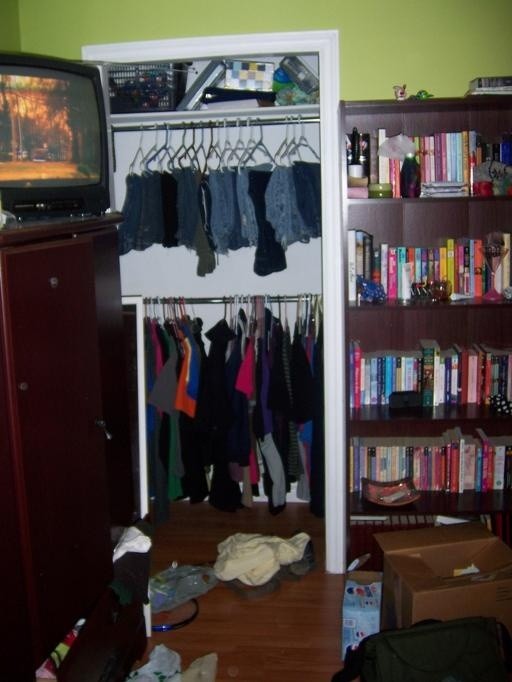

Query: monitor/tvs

(0, 50), (115, 231)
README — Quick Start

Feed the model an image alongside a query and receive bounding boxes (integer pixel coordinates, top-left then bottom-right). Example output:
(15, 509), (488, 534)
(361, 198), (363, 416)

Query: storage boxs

(374, 522), (512, 633)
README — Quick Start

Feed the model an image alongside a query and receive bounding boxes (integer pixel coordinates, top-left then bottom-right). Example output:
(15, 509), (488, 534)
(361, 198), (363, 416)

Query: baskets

(108, 61), (193, 112)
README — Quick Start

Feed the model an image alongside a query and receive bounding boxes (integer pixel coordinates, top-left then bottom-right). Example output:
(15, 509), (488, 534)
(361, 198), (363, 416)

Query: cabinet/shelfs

(338, 96), (512, 575)
(82, 30), (349, 578)
(0, 213), (149, 682)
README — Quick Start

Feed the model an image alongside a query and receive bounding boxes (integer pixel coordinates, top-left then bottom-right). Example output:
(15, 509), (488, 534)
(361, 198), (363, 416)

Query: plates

(362, 477), (421, 509)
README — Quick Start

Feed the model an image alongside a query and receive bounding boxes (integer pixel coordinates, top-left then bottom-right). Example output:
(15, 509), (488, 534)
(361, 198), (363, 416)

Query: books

(345, 126), (512, 200)
(346, 336), (511, 422)
(346, 226), (512, 305)
(348, 426), (511, 576)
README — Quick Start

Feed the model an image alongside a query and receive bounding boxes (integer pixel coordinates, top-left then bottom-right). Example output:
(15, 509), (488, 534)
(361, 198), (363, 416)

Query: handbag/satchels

(337, 617), (511, 681)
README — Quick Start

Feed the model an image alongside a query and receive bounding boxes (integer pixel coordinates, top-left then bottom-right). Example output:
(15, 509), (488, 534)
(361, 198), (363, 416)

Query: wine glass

(478, 245), (508, 302)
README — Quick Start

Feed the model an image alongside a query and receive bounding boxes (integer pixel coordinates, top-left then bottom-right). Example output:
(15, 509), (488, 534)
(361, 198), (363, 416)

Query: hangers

(126, 117), (321, 176)
(145, 294), (323, 343)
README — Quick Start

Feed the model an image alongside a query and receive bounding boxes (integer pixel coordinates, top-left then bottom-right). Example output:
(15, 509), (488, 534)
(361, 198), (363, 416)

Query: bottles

(400, 154), (421, 199)
